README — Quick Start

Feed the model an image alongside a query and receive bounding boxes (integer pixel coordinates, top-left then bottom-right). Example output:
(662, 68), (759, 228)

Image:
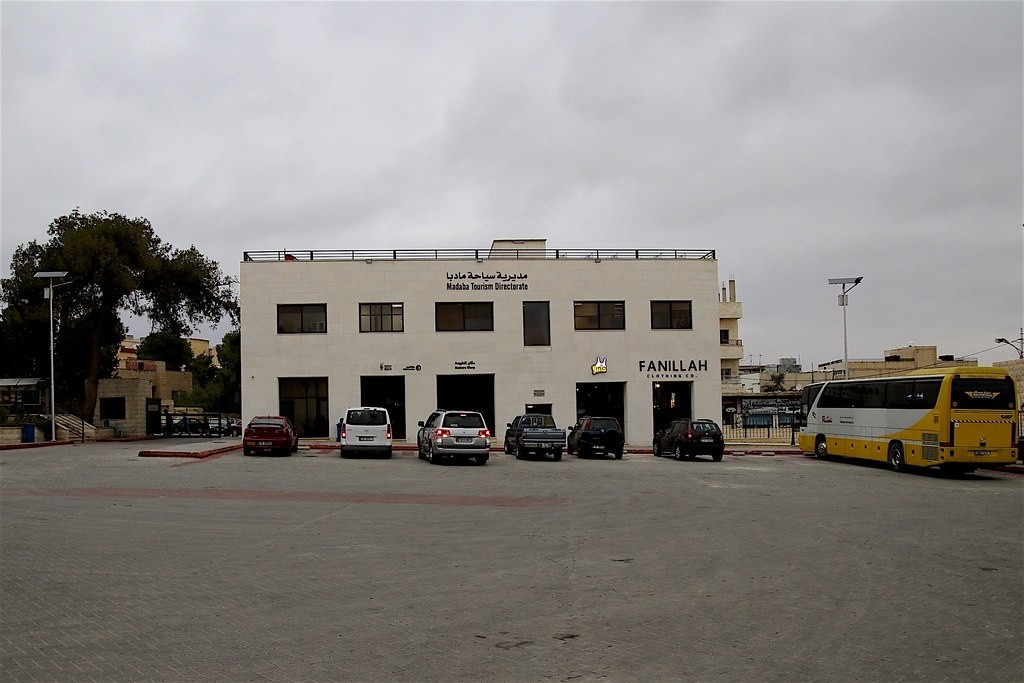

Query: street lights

(34, 272), (72, 441)
(995, 337), (1024, 358)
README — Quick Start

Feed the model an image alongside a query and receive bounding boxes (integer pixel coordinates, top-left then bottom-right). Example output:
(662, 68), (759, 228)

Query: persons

(164, 408), (232, 437)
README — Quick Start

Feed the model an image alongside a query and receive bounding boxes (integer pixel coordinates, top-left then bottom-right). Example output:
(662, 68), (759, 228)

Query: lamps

(595, 259), (601, 263)
(477, 258), (483, 263)
(366, 259), (372, 264)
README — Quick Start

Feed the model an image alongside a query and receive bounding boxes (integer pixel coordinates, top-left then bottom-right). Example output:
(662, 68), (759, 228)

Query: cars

(243, 415), (299, 457)
(161, 417), (242, 437)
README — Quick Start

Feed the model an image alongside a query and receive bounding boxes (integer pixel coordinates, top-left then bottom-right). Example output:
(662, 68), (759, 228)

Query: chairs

(696, 426), (701, 430)
(524, 418), (531, 425)
(703, 427), (709, 431)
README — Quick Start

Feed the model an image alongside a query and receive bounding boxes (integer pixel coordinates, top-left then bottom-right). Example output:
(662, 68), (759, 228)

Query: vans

(341, 406), (393, 459)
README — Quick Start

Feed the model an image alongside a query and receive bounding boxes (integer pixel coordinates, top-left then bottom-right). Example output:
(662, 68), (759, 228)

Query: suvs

(417, 409), (490, 465)
(567, 416), (624, 459)
(652, 418), (725, 462)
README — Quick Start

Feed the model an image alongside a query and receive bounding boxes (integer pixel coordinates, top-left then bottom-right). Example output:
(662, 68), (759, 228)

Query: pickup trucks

(504, 414), (566, 461)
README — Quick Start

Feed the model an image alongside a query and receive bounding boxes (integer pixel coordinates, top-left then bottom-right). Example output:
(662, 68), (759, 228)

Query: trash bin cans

(23, 423), (35, 442)
(336, 418), (344, 442)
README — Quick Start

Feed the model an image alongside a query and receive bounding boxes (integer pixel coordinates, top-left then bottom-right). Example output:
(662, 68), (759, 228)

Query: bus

(745, 407), (806, 429)
(797, 367), (1019, 473)
